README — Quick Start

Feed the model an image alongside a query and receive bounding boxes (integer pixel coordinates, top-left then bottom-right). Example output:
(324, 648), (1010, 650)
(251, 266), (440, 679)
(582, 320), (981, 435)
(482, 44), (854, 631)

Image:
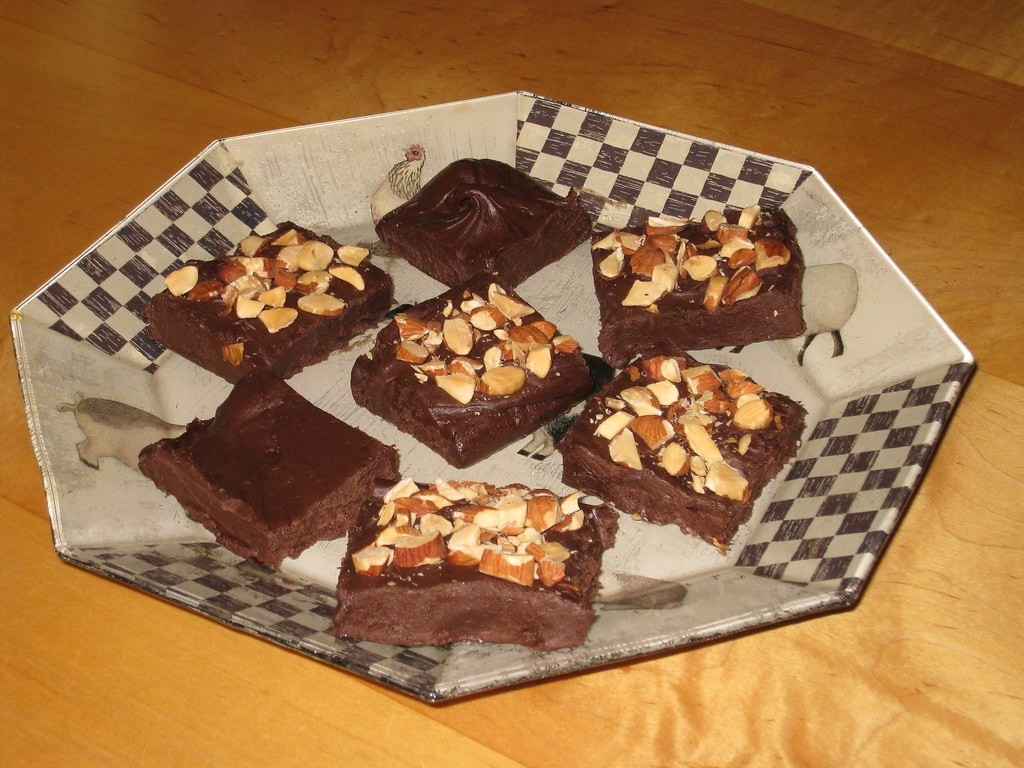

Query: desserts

(136, 155), (809, 651)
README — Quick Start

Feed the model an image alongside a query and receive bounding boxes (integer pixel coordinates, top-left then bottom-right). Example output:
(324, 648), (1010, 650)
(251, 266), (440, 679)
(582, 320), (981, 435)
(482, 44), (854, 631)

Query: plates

(13, 92), (977, 704)
